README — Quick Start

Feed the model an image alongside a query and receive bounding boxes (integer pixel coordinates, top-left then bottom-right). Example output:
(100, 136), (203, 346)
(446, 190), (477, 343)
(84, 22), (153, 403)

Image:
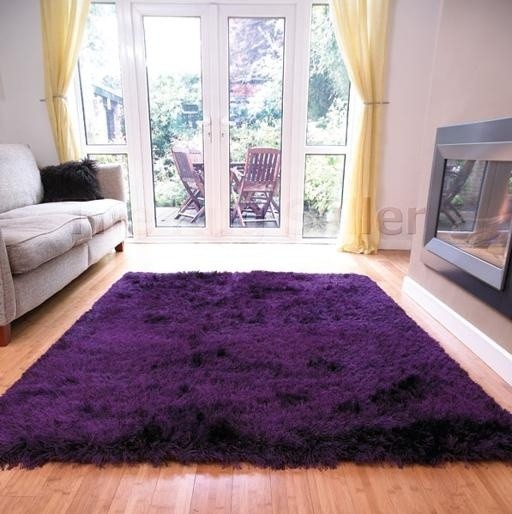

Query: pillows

(38, 154), (104, 203)
(0, 142), (44, 214)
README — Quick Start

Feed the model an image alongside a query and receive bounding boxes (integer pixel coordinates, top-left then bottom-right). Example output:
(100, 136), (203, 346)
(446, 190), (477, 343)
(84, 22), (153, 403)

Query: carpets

(0, 271), (512, 473)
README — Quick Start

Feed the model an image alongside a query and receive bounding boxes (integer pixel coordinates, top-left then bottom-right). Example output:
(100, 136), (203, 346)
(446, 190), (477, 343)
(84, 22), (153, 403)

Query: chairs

(170, 143), (283, 228)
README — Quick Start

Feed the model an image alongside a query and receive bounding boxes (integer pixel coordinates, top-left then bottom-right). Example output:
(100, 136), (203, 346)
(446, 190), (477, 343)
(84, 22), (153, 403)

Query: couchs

(0, 142), (129, 349)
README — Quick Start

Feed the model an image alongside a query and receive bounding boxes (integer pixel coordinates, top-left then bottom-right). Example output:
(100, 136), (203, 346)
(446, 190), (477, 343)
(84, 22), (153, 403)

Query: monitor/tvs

(422, 141), (512, 290)
